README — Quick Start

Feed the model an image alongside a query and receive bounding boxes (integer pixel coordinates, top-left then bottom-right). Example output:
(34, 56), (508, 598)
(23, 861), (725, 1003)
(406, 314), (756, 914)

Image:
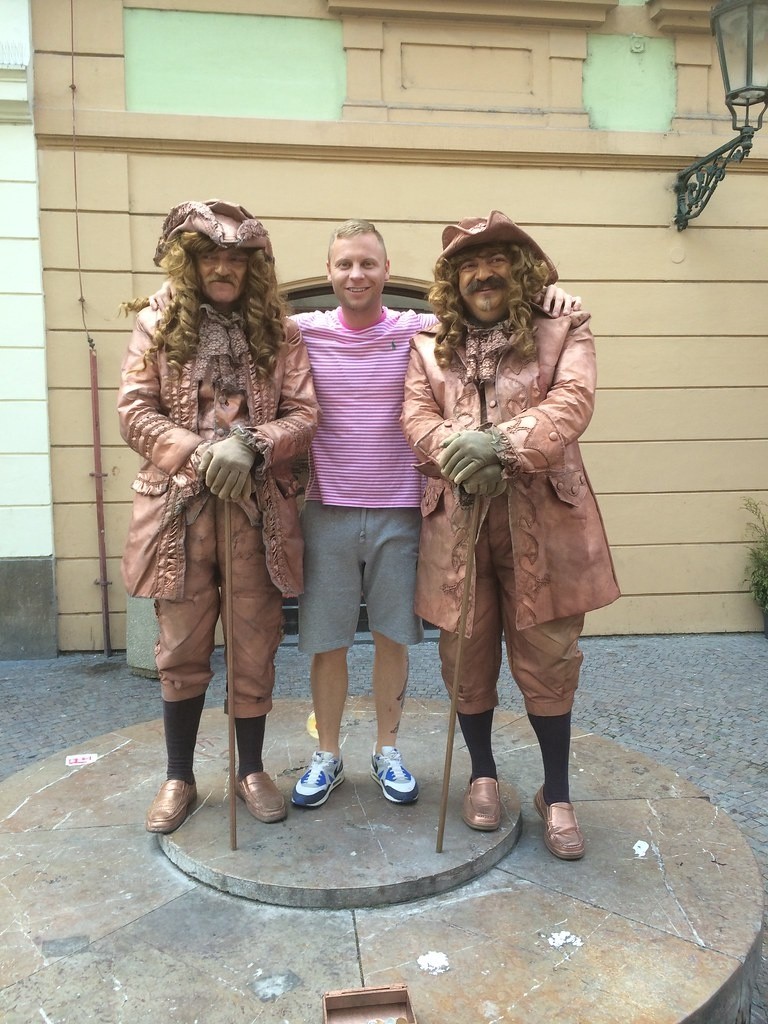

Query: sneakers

(292, 749), (345, 806)
(370, 741), (419, 804)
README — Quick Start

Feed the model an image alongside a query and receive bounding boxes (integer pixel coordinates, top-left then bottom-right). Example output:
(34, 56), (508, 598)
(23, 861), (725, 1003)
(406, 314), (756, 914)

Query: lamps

(674, 0), (768, 232)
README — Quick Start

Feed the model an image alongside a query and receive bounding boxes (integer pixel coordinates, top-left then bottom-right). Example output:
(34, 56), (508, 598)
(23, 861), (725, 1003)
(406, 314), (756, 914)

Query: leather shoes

(146, 779), (196, 833)
(235, 771), (286, 823)
(534, 783), (586, 860)
(461, 775), (500, 831)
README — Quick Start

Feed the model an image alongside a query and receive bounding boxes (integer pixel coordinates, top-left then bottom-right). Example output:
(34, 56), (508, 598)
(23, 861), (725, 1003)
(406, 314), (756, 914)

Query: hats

(435, 211), (558, 288)
(154, 200), (275, 269)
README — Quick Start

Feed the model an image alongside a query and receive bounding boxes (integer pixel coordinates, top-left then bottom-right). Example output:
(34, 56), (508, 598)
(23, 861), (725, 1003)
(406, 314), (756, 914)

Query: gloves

(438, 428), (507, 499)
(191, 425), (257, 504)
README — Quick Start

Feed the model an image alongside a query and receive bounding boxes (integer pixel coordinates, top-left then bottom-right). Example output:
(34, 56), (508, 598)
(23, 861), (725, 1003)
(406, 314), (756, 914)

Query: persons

(399, 209), (623, 862)
(113, 197), (327, 835)
(148, 221), (586, 811)
